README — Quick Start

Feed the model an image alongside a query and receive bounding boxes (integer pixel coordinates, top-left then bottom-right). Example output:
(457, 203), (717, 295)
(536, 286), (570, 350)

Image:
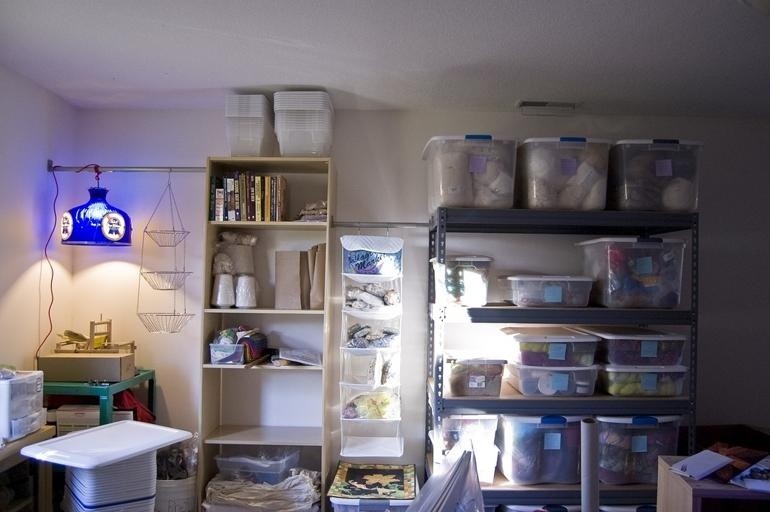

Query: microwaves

(0, 370), (45, 444)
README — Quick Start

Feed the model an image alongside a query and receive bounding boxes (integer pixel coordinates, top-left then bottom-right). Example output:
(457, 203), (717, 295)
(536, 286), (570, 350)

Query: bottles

(234, 272), (258, 309)
(210, 274), (235, 310)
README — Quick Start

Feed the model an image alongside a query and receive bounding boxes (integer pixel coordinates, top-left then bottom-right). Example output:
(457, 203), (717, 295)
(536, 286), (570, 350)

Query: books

(210, 170), (288, 222)
(668, 448), (735, 481)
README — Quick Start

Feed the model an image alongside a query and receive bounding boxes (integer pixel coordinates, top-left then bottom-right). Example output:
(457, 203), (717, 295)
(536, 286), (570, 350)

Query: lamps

(61, 163), (131, 246)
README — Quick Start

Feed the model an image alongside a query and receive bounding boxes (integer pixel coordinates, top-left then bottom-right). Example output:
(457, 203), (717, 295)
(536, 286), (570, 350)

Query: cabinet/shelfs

(339, 235), (405, 457)
(198, 156), (341, 512)
(0, 425), (56, 512)
(656, 455), (770, 512)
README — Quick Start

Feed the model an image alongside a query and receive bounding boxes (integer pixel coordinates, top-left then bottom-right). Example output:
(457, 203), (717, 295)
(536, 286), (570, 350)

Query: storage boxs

(330, 472), (419, 512)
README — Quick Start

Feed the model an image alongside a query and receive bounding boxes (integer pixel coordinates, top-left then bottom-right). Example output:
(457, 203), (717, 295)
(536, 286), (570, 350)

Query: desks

(43, 368), (156, 426)
